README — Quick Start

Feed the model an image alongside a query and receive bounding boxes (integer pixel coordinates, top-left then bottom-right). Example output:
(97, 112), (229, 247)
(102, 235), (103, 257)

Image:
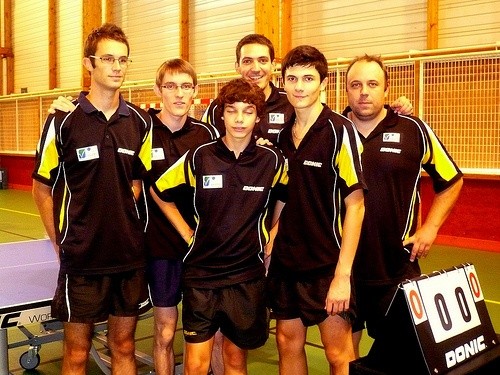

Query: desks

(0, 237), (184, 375)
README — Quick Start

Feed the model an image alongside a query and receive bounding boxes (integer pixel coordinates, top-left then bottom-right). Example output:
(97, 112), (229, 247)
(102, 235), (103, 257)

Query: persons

(201, 34), (413, 143)
(47, 59), (274, 375)
(341, 55), (463, 359)
(33, 23), (151, 375)
(257, 45), (368, 375)
(150, 79), (289, 375)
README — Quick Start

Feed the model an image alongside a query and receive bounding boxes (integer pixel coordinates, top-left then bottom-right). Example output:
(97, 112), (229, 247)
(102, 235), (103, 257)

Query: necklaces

(294, 119), (301, 140)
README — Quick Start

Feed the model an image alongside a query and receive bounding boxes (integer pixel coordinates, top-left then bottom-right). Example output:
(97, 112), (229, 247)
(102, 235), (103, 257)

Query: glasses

(88, 55), (132, 65)
(160, 84), (195, 90)
